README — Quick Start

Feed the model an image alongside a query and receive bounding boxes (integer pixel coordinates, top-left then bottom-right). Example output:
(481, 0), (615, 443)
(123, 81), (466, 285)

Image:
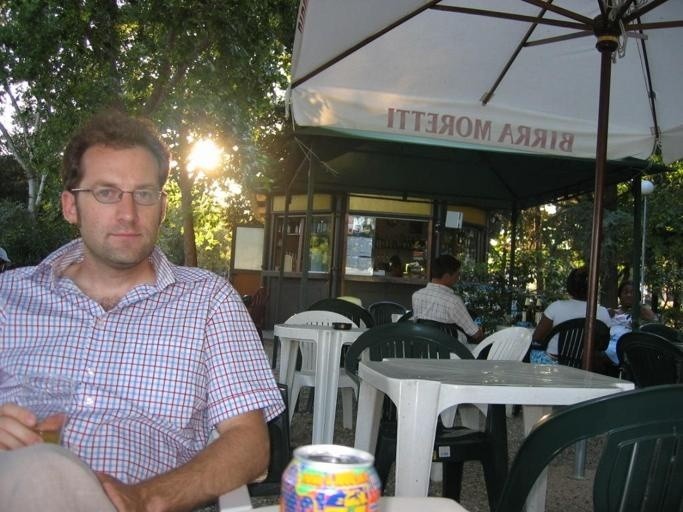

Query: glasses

(72, 186), (162, 205)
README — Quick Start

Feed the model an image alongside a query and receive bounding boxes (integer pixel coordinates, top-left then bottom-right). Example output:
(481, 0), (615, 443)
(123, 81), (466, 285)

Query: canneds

(279, 444), (381, 512)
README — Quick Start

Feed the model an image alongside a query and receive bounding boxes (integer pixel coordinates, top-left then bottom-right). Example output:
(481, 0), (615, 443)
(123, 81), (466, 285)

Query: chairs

(212, 282), (681, 510)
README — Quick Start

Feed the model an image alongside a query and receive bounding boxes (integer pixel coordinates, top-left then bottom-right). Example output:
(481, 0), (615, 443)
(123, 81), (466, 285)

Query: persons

(533, 267), (613, 360)
(606, 282), (661, 364)
(389, 255), (403, 277)
(309, 231), (329, 271)
(412, 255), (485, 349)
(1, 112), (285, 510)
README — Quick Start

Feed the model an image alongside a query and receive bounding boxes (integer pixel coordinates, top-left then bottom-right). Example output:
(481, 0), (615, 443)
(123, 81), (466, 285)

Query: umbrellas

(287, 1), (681, 478)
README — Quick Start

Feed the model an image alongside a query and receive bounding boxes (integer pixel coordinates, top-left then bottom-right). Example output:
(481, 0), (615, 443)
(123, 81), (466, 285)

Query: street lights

(640, 178), (654, 304)
